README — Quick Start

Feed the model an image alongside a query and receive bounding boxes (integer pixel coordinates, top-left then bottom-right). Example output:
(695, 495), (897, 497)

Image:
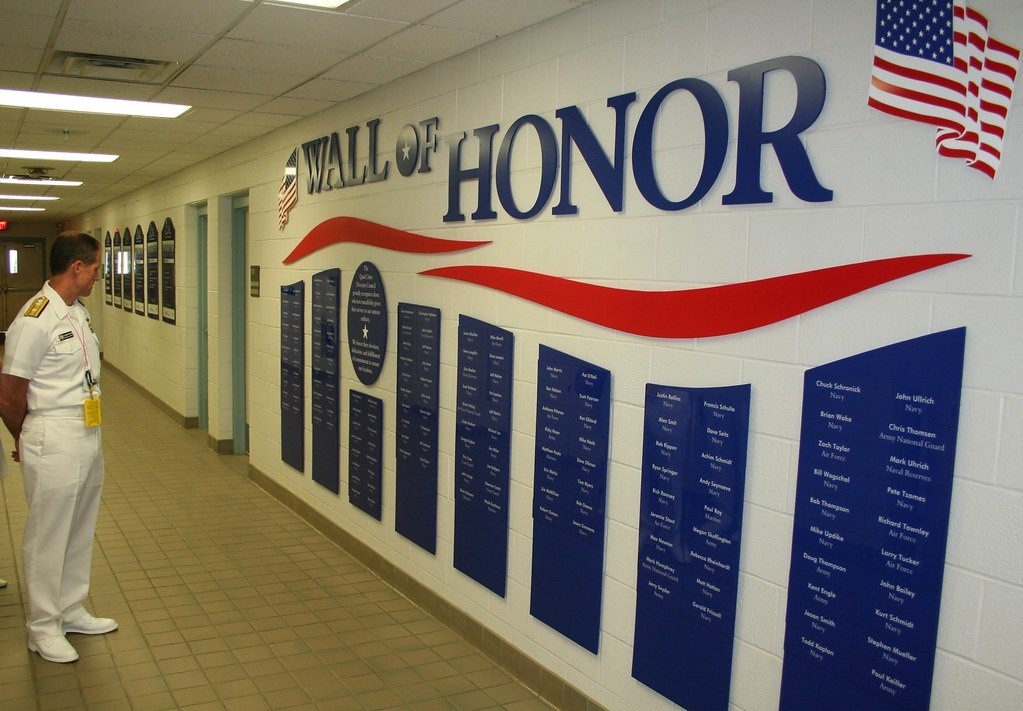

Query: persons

(0, 231), (118, 664)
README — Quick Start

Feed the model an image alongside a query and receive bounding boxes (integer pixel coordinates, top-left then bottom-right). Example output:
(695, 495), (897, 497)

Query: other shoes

(0, 579), (7, 587)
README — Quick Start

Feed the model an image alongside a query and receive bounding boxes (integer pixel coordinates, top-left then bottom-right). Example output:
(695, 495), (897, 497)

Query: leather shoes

(62, 613), (118, 636)
(27, 636), (79, 663)
